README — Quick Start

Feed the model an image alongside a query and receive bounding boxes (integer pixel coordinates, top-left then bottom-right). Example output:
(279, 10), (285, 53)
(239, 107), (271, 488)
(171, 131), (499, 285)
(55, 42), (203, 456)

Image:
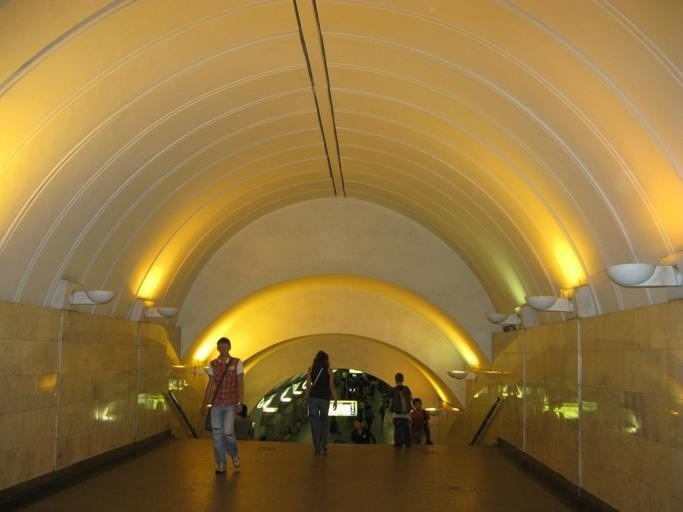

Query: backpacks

(391, 385), (414, 415)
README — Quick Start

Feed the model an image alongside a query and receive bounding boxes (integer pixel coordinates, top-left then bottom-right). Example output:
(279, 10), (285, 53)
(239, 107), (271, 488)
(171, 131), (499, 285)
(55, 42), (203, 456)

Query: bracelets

(236, 401), (242, 405)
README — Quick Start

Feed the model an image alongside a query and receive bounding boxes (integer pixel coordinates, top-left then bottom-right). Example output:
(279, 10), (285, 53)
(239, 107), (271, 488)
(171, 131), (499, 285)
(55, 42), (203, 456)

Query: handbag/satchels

(303, 391), (310, 410)
(205, 407), (212, 431)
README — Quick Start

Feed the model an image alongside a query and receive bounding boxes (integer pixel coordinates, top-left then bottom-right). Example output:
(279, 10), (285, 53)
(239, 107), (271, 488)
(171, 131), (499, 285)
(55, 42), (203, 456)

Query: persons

(329, 371), (433, 448)
(200, 338), (245, 472)
(233, 404), (252, 441)
(302, 349), (338, 454)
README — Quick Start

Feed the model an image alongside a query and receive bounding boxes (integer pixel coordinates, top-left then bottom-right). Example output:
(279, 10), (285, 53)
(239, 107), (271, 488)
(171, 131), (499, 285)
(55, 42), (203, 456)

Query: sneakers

(233, 454), (240, 467)
(216, 464), (226, 473)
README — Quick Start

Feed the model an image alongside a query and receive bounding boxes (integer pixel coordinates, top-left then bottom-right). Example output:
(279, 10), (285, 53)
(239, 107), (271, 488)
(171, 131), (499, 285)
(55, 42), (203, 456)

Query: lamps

(485, 294), (573, 327)
(143, 306), (180, 318)
(605, 261), (681, 290)
(70, 287), (116, 306)
